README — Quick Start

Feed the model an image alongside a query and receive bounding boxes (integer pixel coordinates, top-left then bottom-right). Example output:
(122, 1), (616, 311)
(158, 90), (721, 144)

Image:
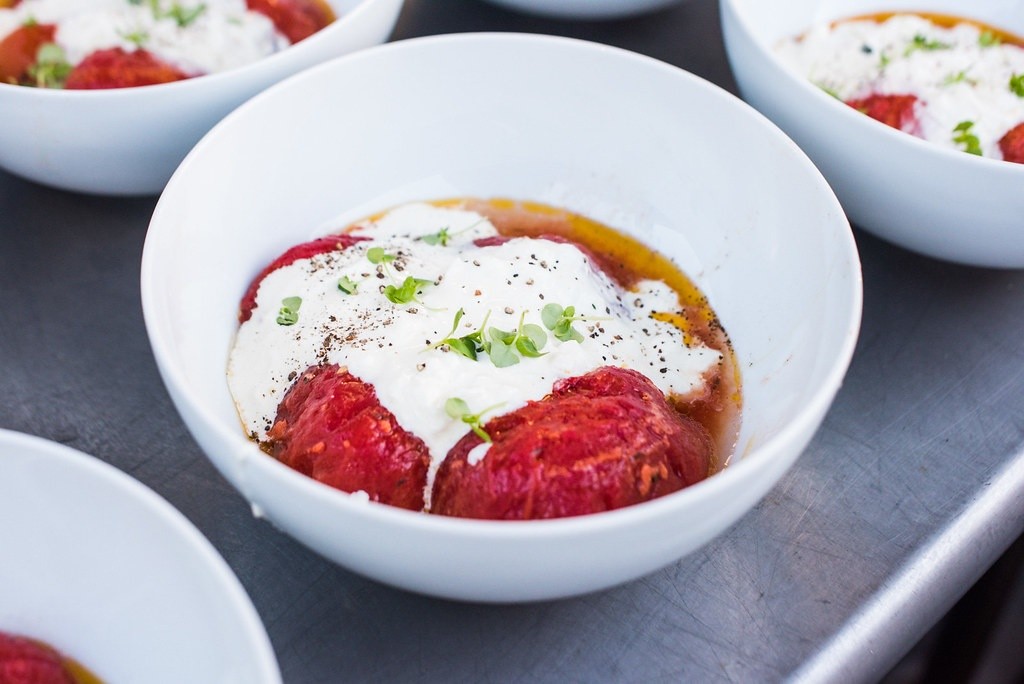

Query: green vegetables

(13, 0), (210, 91)
(817, 32), (1024, 159)
(278, 228), (585, 442)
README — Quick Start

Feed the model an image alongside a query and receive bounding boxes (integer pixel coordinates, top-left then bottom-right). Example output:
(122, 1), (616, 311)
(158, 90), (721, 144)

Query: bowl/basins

(714, 0), (1024, 272)
(0, 0), (408, 197)
(138, 31), (866, 603)
(0, 429), (285, 684)
